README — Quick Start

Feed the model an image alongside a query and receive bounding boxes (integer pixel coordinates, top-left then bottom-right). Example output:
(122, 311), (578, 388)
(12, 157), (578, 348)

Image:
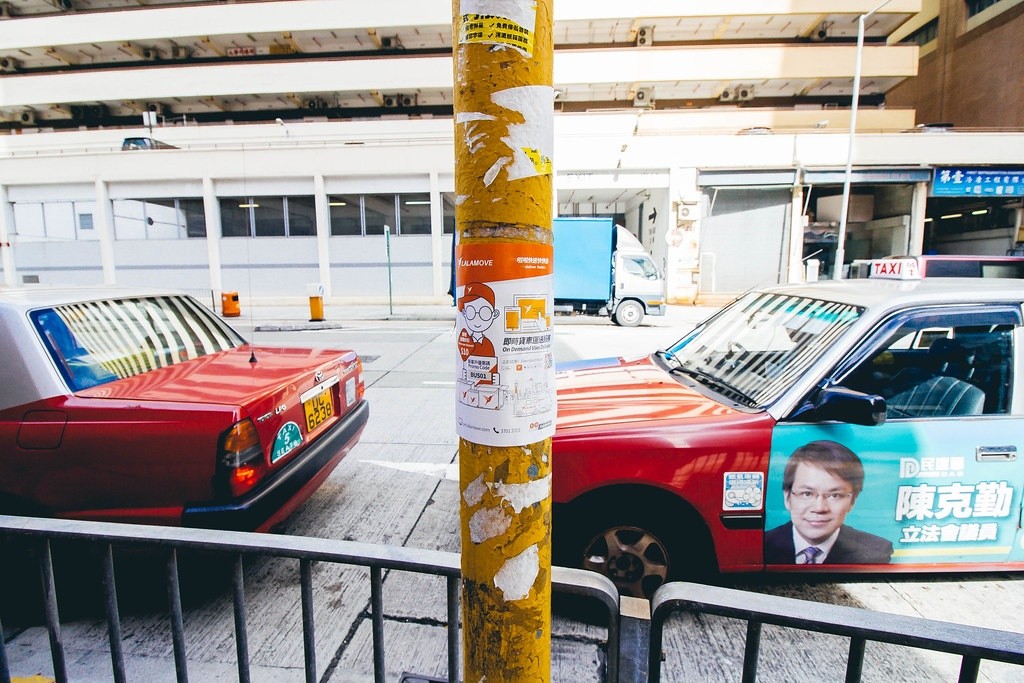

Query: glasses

(790, 489), (854, 502)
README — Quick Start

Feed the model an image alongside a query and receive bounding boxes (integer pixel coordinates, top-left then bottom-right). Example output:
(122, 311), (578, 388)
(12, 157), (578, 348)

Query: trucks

(554, 215), (670, 327)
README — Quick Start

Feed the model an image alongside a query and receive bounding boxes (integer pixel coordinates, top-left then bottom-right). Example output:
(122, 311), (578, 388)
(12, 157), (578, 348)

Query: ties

(800, 545), (822, 565)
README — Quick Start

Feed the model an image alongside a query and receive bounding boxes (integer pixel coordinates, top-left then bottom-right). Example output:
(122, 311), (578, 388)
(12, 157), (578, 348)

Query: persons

(764, 441), (893, 564)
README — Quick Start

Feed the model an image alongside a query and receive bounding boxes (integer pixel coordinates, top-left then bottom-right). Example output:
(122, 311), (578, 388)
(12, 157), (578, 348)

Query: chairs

(880, 338), (985, 418)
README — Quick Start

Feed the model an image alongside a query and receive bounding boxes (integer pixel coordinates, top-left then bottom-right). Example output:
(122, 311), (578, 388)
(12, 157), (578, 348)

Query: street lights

(832, 0), (892, 276)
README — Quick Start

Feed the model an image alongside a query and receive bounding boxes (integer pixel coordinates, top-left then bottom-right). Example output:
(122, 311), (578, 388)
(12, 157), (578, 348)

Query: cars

(544, 276), (1024, 609)
(0, 290), (371, 608)
